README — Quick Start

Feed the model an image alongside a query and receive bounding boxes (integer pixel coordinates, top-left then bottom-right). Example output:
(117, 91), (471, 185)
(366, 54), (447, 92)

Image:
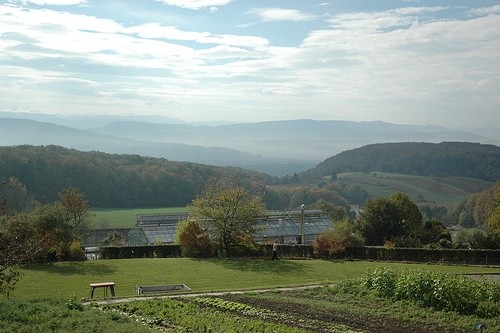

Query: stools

(89, 281), (116, 299)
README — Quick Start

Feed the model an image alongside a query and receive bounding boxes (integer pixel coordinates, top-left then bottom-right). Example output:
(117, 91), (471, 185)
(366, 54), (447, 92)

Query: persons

(271, 240), (279, 261)
